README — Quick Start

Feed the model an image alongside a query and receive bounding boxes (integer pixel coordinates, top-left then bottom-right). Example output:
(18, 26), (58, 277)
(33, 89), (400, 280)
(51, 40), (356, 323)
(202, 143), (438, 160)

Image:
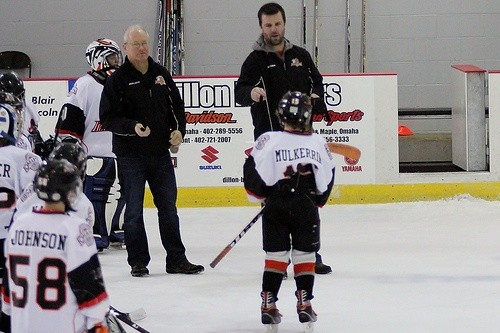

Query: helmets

(34, 158), (83, 205)
(0, 104), (19, 144)
(49, 143), (86, 181)
(277, 90), (313, 125)
(0, 71), (25, 97)
(0, 91), (23, 110)
(85, 38), (123, 76)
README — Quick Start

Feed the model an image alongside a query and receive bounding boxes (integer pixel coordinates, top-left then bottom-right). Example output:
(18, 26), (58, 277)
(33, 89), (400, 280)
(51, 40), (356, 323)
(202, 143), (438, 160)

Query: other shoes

(315, 261), (332, 274)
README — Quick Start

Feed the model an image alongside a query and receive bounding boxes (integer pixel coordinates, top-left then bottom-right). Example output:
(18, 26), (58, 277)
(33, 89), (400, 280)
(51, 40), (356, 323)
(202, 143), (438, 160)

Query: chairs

(0, 51), (31, 79)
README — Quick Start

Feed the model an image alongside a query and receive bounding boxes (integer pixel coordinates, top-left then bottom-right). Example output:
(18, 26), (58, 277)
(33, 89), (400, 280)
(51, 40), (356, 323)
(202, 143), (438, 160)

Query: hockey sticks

(157, 0), (185, 75)
(314, 0), (319, 69)
(346, 0), (351, 73)
(303, 0), (307, 50)
(110, 305), (149, 333)
(210, 206), (264, 268)
(362, 0), (366, 73)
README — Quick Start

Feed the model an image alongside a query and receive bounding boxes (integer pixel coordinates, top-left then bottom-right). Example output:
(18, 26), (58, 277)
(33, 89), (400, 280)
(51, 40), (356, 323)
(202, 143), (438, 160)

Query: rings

(177, 140), (180, 143)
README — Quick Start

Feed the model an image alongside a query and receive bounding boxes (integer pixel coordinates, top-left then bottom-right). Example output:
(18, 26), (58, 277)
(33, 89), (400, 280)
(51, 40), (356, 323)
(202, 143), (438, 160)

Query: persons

(0, 69), (115, 333)
(235, 2), (333, 281)
(243, 92), (336, 333)
(99, 24), (206, 278)
(66, 37), (129, 253)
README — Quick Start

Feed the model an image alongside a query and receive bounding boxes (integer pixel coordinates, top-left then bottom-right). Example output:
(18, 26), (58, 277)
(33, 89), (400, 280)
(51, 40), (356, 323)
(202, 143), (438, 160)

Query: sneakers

(166, 258), (204, 274)
(259, 290), (282, 332)
(131, 263), (149, 277)
(294, 289), (317, 333)
